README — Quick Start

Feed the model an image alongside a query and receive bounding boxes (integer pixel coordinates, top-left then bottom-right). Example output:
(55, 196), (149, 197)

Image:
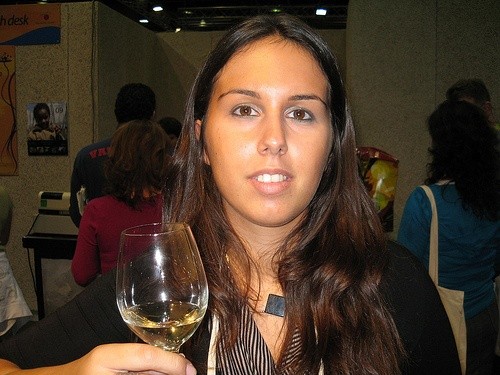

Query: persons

(397, 99), (500, 373)
(447, 78), (495, 122)
(71, 120), (172, 285)
(0, 15), (464, 375)
(30, 102), (64, 139)
(69, 83), (185, 227)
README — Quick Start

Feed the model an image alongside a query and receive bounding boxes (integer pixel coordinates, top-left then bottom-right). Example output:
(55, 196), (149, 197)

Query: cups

(115, 223), (208, 353)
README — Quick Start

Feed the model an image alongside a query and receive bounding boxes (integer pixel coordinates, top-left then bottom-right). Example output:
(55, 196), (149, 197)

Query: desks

(22, 213), (80, 321)
